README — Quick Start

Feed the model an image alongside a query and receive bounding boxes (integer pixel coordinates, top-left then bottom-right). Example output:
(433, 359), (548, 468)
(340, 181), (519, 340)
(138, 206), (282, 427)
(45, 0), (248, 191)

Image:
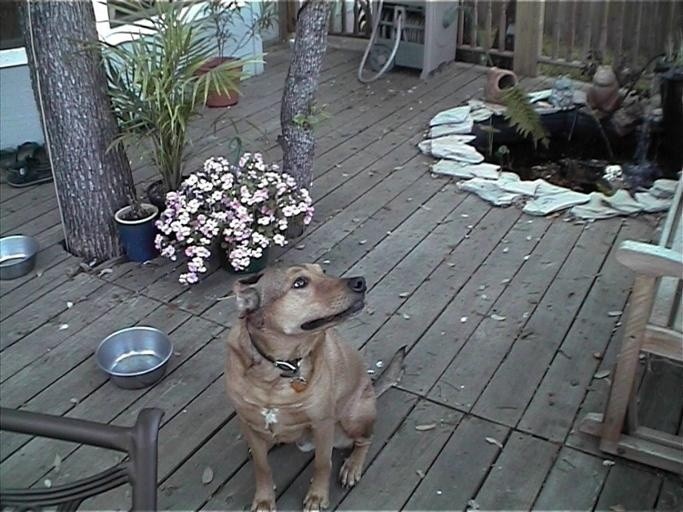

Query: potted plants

(61, 1), (276, 264)
(440, 2), (519, 104)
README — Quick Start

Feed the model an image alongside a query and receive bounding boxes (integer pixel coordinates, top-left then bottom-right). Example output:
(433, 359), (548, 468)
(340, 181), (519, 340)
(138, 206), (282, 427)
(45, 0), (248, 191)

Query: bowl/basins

(95, 326), (175, 390)
(0, 236), (40, 281)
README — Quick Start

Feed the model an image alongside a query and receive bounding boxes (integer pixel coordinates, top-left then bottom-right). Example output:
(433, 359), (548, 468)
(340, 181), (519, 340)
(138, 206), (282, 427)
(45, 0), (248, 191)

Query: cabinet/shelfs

(576, 182), (682, 472)
(370, 2), (460, 81)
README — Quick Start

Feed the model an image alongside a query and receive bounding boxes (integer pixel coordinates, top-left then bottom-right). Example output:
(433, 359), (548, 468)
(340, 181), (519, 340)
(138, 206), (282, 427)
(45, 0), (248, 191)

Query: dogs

(220, 257), (410, 512)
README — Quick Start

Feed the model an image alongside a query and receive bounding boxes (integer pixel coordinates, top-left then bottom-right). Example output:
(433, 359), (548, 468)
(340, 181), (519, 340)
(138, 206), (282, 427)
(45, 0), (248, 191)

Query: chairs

(0, 407), (166, 511)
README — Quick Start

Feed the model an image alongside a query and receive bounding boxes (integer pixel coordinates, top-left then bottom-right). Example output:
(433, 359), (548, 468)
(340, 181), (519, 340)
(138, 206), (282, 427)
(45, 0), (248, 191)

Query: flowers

(151, 149), (315, 285)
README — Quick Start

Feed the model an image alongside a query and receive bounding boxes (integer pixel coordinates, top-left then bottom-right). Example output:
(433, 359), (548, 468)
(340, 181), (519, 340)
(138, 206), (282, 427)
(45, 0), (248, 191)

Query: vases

(217, 236), (269, 274)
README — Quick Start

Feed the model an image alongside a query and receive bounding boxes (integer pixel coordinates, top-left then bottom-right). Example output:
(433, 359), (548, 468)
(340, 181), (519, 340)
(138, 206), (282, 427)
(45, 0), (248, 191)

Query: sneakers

(7, 155), (53, 187)
